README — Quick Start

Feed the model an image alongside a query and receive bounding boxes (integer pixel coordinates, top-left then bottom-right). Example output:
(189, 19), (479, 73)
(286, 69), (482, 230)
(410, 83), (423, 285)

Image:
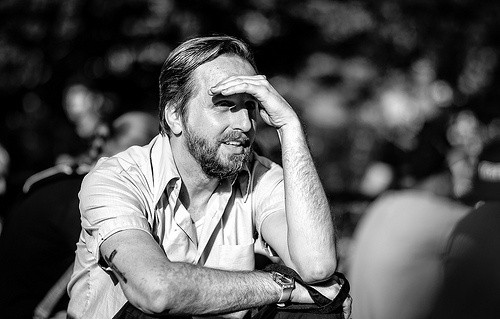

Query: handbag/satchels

(242, 262), (351, 319)
(111, 300), (193, 319)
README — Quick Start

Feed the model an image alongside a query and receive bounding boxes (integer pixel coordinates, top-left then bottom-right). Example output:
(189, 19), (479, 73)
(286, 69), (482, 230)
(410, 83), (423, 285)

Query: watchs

(268, 268), (294, 308)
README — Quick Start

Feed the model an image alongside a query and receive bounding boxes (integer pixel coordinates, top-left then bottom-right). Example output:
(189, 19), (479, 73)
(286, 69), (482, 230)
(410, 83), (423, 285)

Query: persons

(433, 198), (500, 319)
(347, 151), (477, 319)
(65, 37), (353, 319)
